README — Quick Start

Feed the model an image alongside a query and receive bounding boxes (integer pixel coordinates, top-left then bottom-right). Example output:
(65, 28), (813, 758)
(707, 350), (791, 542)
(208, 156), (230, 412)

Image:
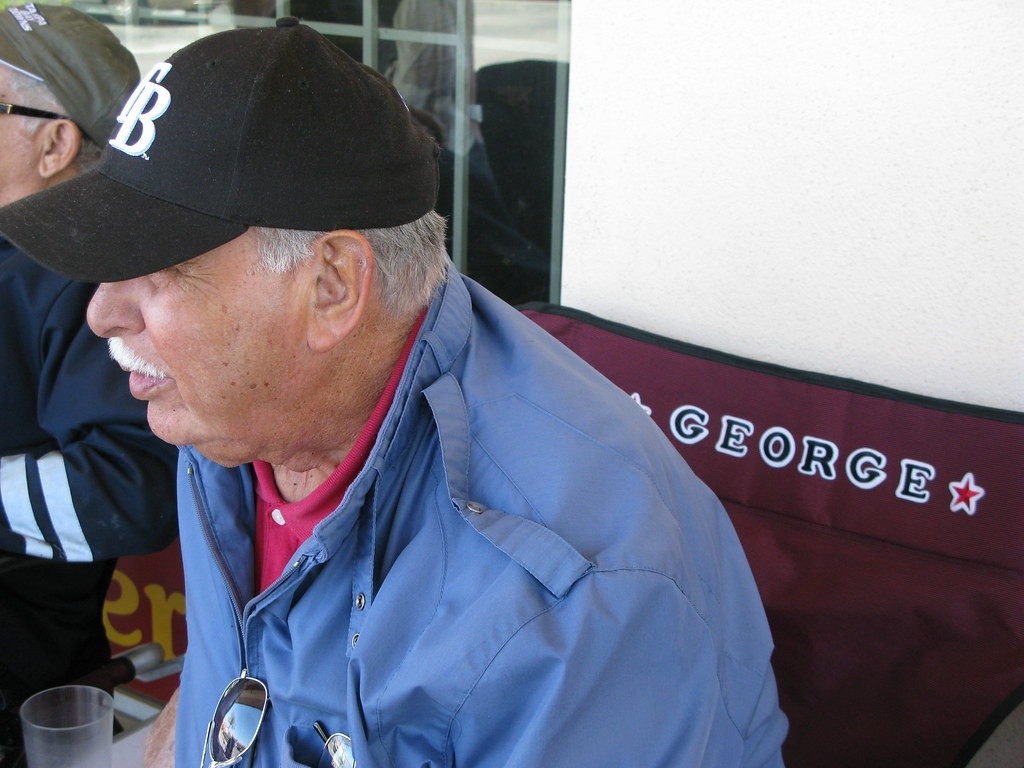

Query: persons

(0, 20), (791, 768)
(0, 6), (179, 767)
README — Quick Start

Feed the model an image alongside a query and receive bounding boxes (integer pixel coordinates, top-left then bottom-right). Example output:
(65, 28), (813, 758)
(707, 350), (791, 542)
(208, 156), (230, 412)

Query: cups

(19, 684), (115, 768)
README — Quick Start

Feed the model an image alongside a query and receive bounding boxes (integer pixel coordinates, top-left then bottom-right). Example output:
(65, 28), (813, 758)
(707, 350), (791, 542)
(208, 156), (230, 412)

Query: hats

(0, 16), (438, 282)
(0, 4), (141, 149)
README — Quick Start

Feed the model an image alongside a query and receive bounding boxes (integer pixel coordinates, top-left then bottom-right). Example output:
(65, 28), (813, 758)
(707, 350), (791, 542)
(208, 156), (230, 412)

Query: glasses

(199, 668), (269, 768)
(313, 722), (356, 768)
